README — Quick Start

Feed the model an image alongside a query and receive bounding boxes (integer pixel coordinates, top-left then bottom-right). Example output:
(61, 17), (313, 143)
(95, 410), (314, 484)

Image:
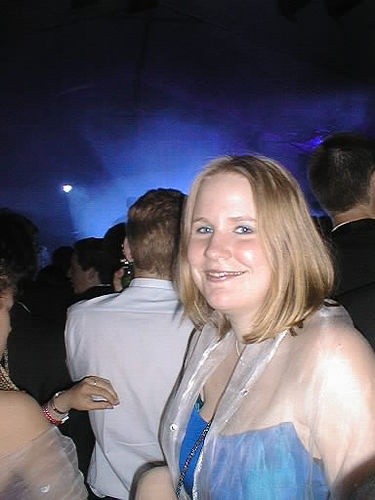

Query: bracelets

(42, 391), (69, 426)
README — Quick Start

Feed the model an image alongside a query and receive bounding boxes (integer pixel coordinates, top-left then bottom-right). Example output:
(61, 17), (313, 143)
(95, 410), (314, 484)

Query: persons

(308, 131), (375, 348)
(0, 210), (49, 405)
(34, 220), (135, 472)
(64, 188), (207, 500)
(132, 152), (375, 500)
(0, 260), (120, 499)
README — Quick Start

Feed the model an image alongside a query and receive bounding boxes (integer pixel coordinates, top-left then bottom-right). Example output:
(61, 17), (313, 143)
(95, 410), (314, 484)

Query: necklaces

(235, 339), (246, 367)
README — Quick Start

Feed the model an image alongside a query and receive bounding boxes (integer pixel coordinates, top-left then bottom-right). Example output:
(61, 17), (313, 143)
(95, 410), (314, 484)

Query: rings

(93, 379), (97, 387)
